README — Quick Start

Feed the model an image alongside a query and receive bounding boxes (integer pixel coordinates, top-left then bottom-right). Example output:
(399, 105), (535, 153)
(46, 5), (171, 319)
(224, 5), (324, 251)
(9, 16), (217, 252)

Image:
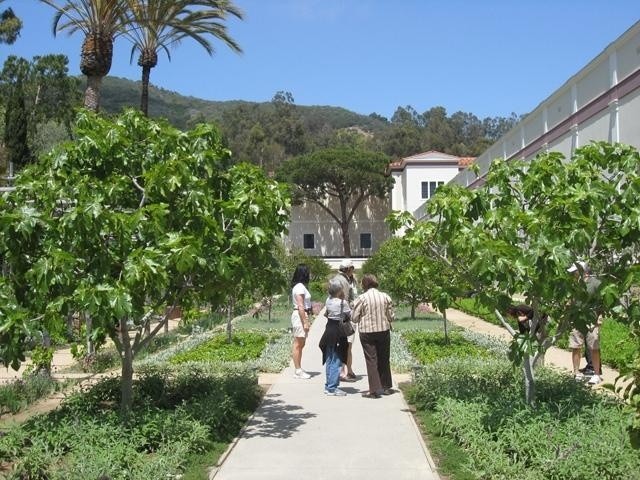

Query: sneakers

(571, 366), (602, 385)
(339, 373), (357, 381)
(323, 387), (347, 396)
(295, 369), (311, 380)
(362, 387), (393, 398)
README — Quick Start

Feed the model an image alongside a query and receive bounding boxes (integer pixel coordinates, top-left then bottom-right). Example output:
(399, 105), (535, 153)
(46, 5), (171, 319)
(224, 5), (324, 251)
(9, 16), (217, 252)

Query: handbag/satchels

(339, 319), (355, 337)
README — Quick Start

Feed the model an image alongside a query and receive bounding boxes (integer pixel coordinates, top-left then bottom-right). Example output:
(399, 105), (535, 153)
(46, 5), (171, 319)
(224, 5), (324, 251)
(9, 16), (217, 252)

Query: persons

(566, 259), (605, 385)
(290, 263), (313, 379)
(514, 304), (547, 335)
(578, 268), (604, 376)
(322, 278), (353, 398)
(328, 260), (360, 382)
(350, 271), (396, 399)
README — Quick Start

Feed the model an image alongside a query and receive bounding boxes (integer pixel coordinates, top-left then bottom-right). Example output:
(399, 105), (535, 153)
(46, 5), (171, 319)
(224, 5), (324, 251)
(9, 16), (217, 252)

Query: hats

(340, 260), (354, 268)
(566, 261), (587, 272)
